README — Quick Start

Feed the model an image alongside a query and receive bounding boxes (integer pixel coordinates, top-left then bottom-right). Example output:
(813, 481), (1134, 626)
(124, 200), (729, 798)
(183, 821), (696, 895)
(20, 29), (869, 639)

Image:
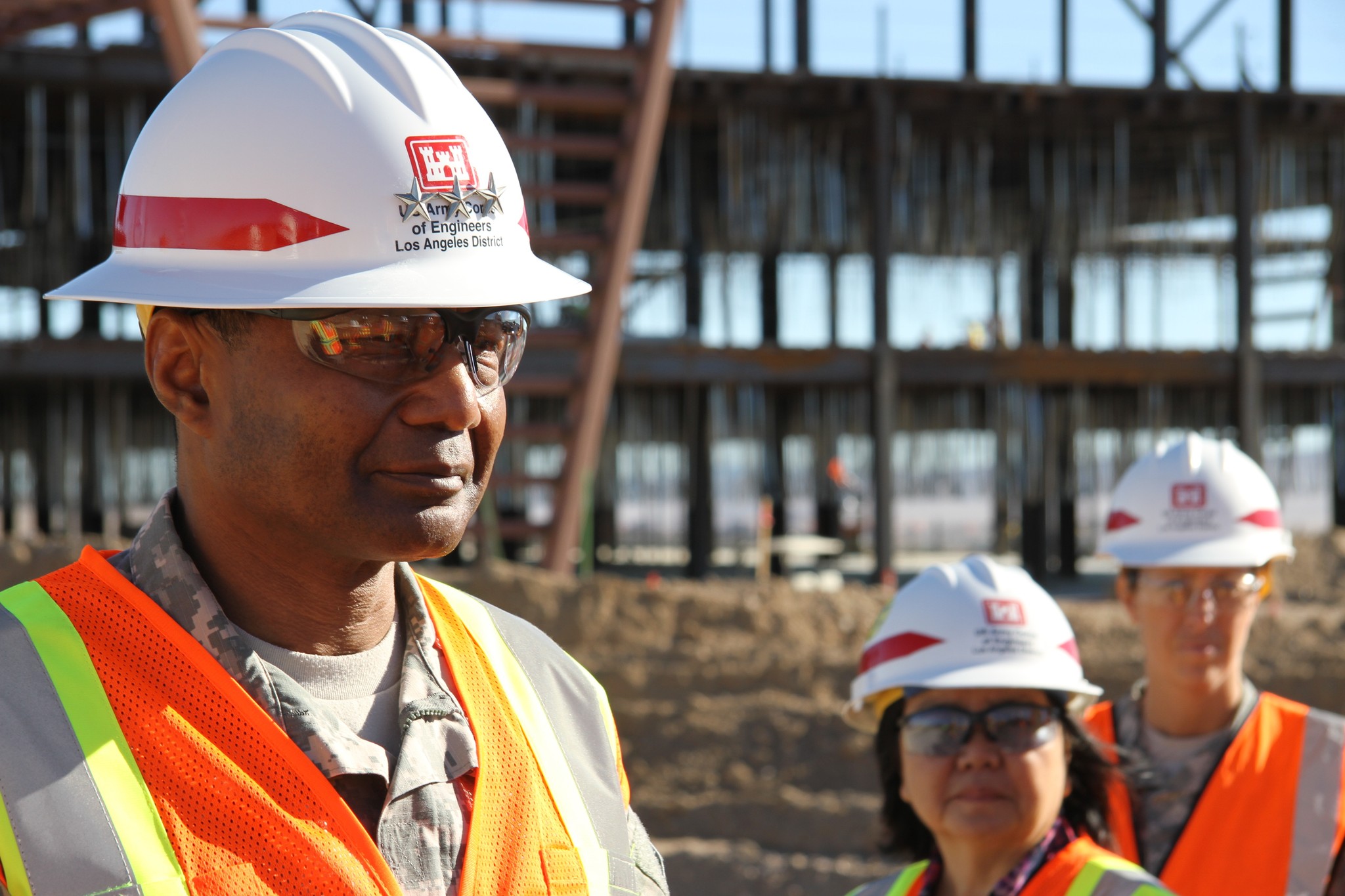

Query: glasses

(239, 305), (532, 389)
(895, 701), (1064, 756)
(1131, 574), (1265, 610)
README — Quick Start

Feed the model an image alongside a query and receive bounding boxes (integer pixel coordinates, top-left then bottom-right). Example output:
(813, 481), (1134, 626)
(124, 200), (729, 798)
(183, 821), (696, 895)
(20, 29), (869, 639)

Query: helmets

(43, 12), (592, 309)
(1098, 431), (1298, 568)
(842, 555), (1105, 735)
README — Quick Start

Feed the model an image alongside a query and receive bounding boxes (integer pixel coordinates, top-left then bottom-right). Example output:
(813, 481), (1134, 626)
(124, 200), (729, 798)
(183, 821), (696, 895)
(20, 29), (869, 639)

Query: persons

(841, 553), (1176, 896)
(1079, 430), (1344, 896)
(0, 11), (671, 896)
